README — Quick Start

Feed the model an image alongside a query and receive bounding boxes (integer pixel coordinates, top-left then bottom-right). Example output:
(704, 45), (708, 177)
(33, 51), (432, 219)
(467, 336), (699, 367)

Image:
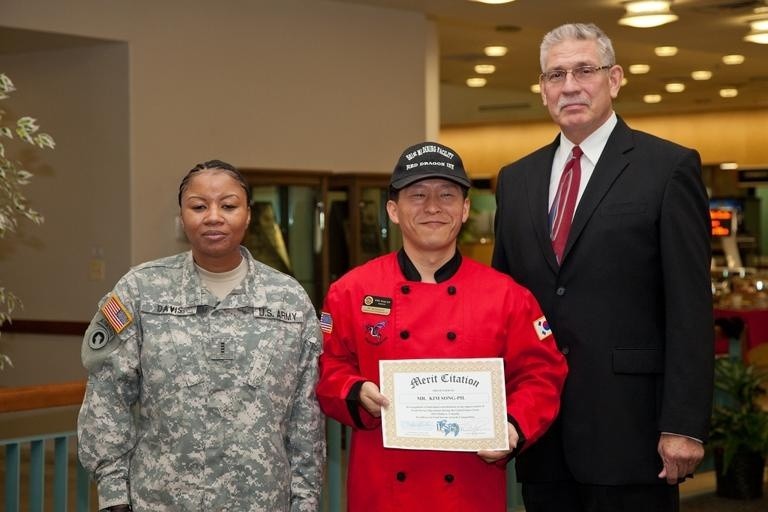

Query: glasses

(542, 65), (612, 83)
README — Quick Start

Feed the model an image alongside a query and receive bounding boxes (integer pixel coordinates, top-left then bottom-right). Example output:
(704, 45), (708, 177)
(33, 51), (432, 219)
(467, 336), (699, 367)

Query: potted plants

(705, 350), (768, 501)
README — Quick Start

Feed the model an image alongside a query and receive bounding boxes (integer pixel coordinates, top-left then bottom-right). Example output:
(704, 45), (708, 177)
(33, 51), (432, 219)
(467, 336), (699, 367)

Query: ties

(549, 146), (584, 265)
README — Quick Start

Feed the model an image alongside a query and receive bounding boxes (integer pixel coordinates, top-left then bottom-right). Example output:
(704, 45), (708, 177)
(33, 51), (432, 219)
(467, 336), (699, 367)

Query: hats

(389, 141), (472, 188)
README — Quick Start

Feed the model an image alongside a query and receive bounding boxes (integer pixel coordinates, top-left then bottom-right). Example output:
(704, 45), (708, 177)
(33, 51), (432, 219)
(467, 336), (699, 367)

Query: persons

(487, 21), (714, 512)
(76, 157), (329, 512)
(311, 140), (568, 512)
